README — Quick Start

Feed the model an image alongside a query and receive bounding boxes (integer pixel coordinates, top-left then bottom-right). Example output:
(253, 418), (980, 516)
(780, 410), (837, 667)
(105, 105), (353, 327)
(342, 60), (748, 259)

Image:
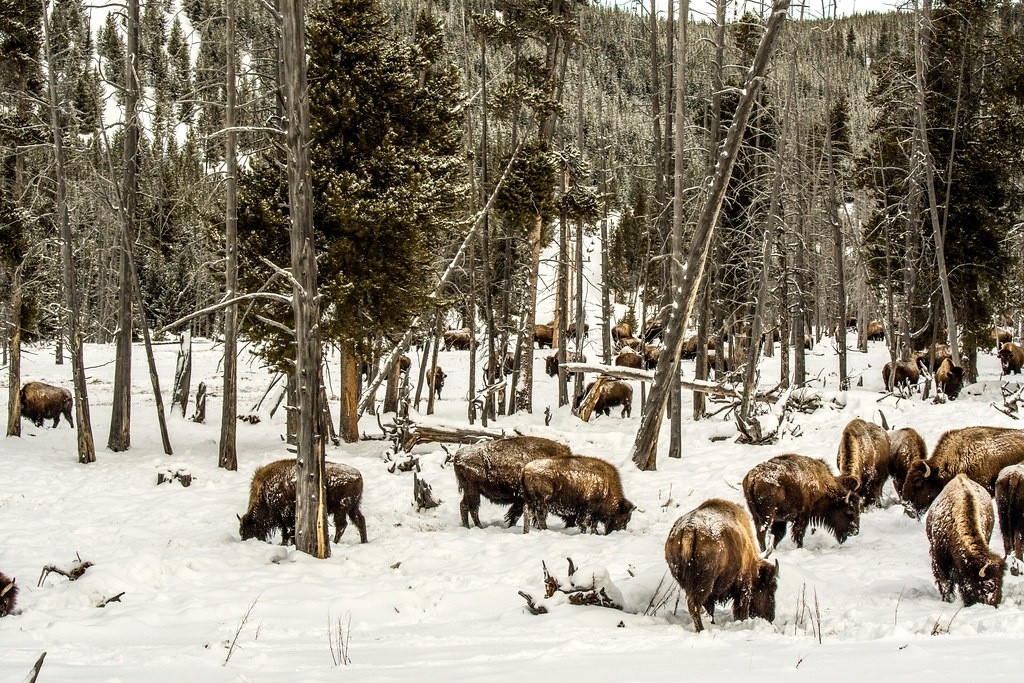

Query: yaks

(742, 454), (861, 553)
(925, 473), (1008, 609)
(836, 419), (893, 514)
(664, 497), (779, 632)
(237, 459), (368, 546)
(520, 455), (638, 534)
(876, 424), (927, 501)
(384, 315), (1023, 419)
(18, 381), (74, 429)
(995, 460), (1024, 561)
(454, 436), (578, 529)
(902, 426), (1024, 512)
